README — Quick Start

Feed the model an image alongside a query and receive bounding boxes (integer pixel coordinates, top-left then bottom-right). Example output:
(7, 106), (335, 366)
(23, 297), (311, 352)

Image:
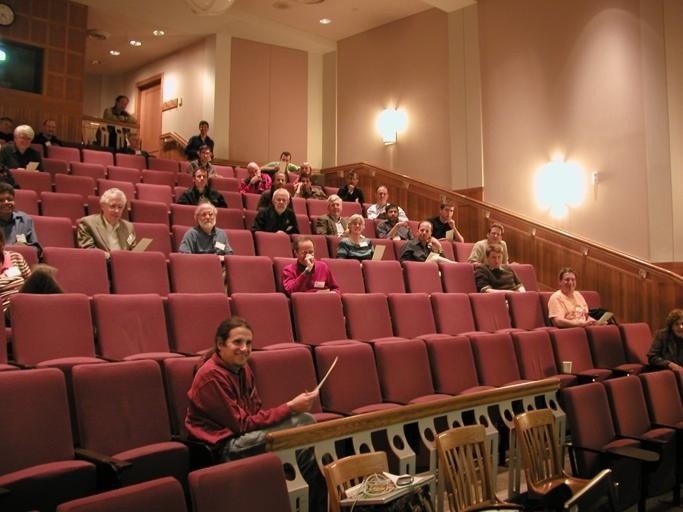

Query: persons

(1, 164), (19, 193)
(76, 189), (136, 282)
(1, 117), (15, 142)
(475, 244), (526, 292)
(646, 308), (683, 384)
(186, 145), (220, 178)
(184, 121), (214, 161)
(238, 151), (464, 264)
(1, 125), (43, 176)
(280, 236), (341, 298)
(0, 184), (37, 247)
(176, 169), (227, 208)
(177, 202), (235, 254)
(184, 316), (331, 512)
(468, 223), (509, 264)
(94, 94), (139, 150)
(0, 230), (62, 328)
(547, 266), (609, 328)
(30, 120), (62, 152)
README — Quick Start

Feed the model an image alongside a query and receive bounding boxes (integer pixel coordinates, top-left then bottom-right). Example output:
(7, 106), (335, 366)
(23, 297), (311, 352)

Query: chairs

(558, 384), (671, 512)
(558, 467), (622, 512)
(638, 370), (682, 447)
(512, 404), (624, 512)
(599, 374), (681, 509)
(432, 423), (527, 512)
(480, 254), (653, 468)
(322, 450), (391, 511)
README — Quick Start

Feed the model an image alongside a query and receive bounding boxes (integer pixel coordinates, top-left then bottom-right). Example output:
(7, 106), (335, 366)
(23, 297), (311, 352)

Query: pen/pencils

(303, 387), (310, 396)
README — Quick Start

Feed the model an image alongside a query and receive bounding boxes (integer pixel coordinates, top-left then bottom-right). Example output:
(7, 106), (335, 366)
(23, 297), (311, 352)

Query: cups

(562, 361), (571, 374)
